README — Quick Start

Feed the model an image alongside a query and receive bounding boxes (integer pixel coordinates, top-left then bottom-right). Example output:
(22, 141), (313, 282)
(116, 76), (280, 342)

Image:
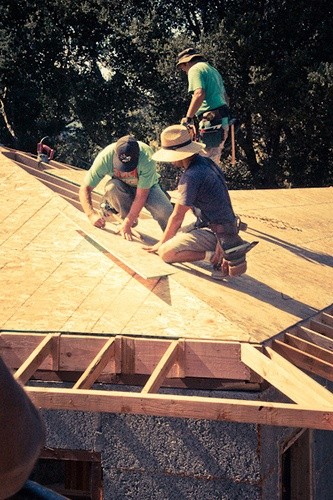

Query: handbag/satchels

(200, 125), (226, 149)
(210, 232), (250, 278)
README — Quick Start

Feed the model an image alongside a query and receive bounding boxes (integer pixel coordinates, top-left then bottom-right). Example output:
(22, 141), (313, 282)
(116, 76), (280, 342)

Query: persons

(177, 48), (229, 165)
(142, 124), (247, 270)
(78, 135), (174, 241)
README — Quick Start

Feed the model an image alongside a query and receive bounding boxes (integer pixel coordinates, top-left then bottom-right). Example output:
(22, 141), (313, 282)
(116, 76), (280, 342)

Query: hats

(152, 125), (206, 162)
(113, 135), (140, 172)
(176, 47), (205, 67)
